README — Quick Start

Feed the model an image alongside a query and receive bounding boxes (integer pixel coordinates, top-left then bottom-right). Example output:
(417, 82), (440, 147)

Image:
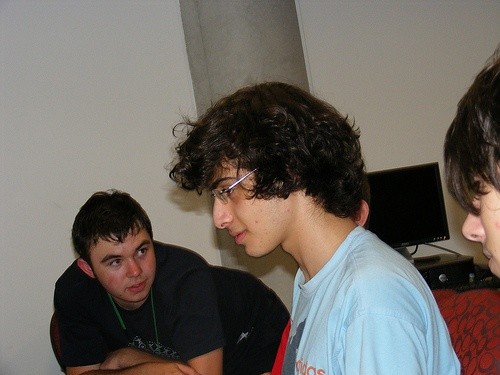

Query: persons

(444, 48), (500, 283)
(59, 189), (224, 375)
(169, 79), (461, 375)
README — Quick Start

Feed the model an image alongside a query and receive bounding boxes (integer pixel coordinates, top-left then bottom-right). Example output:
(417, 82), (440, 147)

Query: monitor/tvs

(366, 162), (450, 264)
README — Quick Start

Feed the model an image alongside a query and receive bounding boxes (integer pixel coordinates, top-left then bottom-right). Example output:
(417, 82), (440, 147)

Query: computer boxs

(414, 253), (475, 291)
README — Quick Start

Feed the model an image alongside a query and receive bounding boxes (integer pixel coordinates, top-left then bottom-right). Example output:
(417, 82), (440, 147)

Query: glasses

(208, 169), (258, 205)
